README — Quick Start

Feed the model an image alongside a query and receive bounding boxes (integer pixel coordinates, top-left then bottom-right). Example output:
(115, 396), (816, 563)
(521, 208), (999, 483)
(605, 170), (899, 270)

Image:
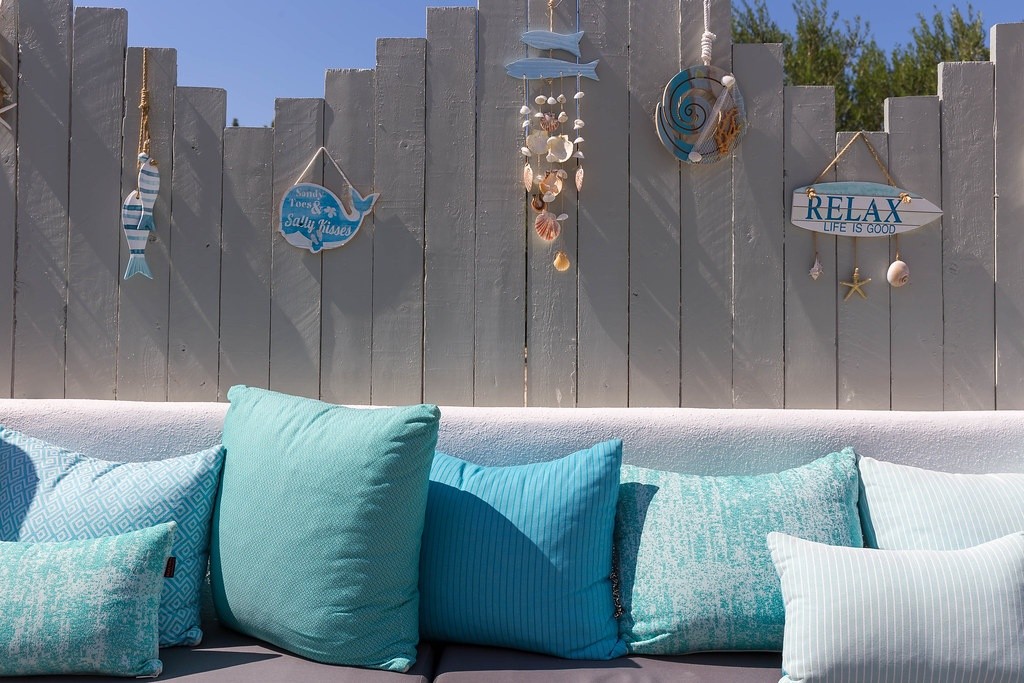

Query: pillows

(214, 385), (443, 674)
(767, 527), (1024, 683)
(855, 451), (1024, 549)
(1, 424), (228, 647)
(610, 446), (863, 657)
(422, 438), (631, 662)
(0, 520), (176, 679)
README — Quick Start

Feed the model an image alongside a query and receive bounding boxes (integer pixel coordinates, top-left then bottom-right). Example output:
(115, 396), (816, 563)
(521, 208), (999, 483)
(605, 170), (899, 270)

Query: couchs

(0, 399), (1024, 683)
